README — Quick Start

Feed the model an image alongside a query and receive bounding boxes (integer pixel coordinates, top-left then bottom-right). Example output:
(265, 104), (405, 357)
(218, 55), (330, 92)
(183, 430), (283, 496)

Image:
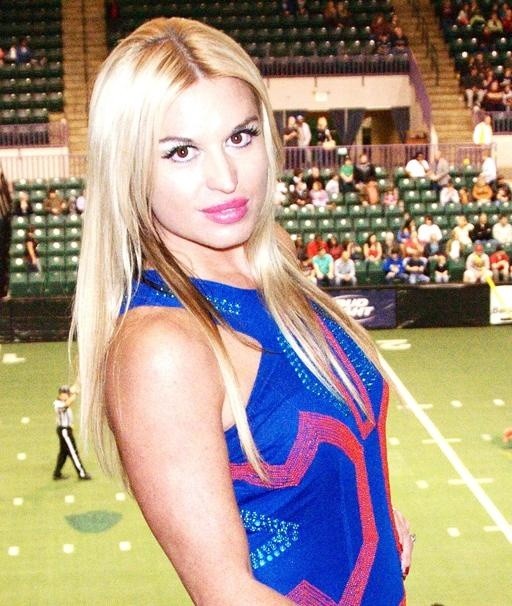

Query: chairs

(280, 164), (511, 285)
(438, 3), (510, 128)
(7, 177), (85, 298)
(2, 1), (65, 124)
(107, 2), (409, 73)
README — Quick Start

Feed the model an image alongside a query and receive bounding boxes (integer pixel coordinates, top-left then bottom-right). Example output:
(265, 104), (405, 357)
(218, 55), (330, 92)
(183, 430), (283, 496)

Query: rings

(410, 534), (414, 543)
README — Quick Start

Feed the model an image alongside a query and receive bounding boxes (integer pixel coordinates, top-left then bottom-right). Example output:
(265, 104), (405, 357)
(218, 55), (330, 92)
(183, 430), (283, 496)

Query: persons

(54, 385), (90, 481)
(68, 16), (415, 606)
